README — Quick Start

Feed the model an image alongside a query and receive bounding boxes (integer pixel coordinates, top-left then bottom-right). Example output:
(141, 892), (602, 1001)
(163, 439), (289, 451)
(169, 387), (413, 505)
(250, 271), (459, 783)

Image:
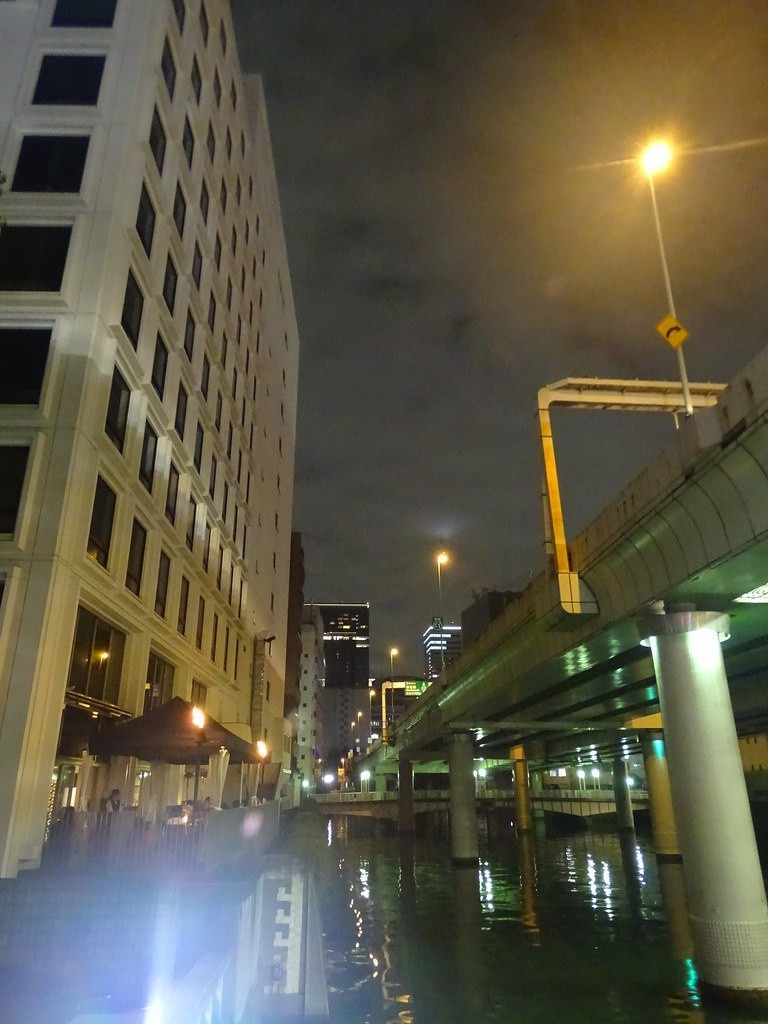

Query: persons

(180, 797), (248, 830)
(104, 788), (122, 834)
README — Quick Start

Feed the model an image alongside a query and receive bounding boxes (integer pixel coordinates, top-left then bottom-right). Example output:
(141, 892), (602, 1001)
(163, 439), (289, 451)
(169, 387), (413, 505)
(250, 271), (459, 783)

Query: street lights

(390, 649), (399, 723)
(189, 705), (205, 877)
(640, 140), (695, 416)
(358, 712), (362, 756)
(369, 691), (375, 745)
(351, 722), (355, 758)
(256, 739), (268, 805)
(437, 551), (450, 669)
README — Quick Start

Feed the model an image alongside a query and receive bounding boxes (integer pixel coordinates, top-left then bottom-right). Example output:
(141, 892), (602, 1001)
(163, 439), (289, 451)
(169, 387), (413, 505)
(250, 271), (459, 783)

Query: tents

(86, 695), (262, 829)
